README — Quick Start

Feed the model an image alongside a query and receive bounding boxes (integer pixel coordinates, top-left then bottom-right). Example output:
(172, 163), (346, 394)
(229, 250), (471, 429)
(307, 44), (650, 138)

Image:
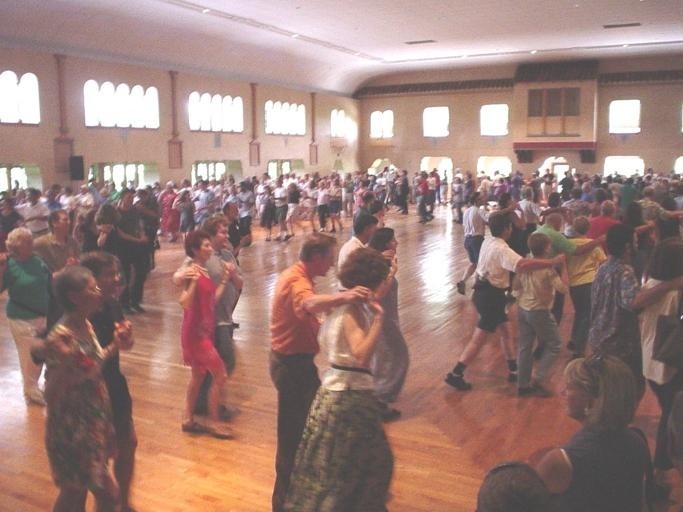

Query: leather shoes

(382, 409), (400, 421)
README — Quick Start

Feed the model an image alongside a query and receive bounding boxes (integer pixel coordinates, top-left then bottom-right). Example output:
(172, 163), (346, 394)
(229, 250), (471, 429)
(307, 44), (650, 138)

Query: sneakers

(444, 372), (471, 390)
(457, 281), (465, 296)
(509, 373), (550, 397)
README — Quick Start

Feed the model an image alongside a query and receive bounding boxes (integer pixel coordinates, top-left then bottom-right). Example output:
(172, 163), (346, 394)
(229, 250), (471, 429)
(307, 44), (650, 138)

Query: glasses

(583, 350), (605, 409)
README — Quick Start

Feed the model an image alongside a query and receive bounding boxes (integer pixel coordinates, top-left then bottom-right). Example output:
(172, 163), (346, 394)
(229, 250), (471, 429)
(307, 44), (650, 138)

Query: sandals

(182, 422), (213, 433)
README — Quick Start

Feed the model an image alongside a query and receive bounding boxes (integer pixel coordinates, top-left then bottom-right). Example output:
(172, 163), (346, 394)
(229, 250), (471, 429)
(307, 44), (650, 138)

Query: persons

(446, 166), (681, 470)
(0, 166), (449, 315)
(172, 216), (245, 436)
(32, 252), (139, 512)
(476, 352), (682, 510)
(0, 228), (52, 404)
(268, 212), (410, 511)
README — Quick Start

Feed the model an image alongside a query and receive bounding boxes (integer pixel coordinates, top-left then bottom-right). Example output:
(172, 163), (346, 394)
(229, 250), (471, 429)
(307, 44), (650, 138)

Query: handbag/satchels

(651, 287), (683, 369)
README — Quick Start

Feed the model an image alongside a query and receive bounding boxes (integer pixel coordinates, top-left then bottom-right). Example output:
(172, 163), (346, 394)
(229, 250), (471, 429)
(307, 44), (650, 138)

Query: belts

(478, 277), (487, 282)
(330, 363), (374, 377)
